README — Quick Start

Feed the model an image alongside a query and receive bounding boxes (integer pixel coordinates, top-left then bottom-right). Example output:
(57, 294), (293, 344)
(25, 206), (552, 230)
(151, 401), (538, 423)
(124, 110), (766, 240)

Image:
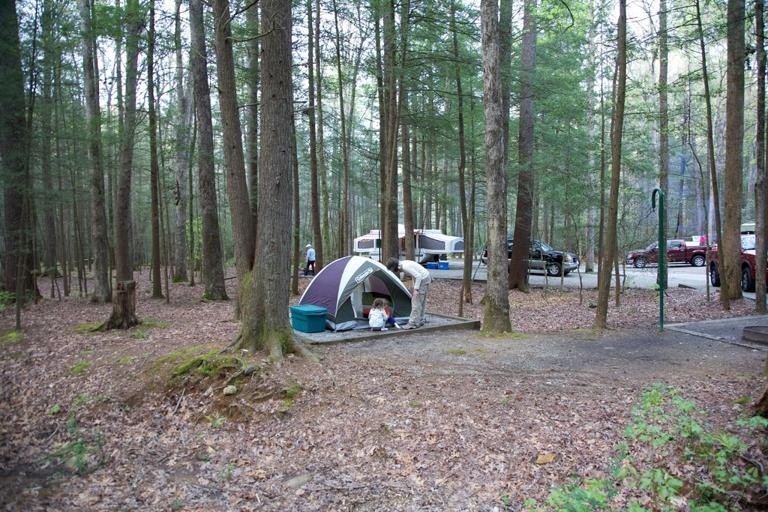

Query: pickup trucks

(625, 239), (708, 268)
(705, 233), (768, 294)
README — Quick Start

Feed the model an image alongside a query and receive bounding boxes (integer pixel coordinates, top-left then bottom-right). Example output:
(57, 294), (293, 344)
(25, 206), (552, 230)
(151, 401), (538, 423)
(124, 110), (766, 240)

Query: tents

(299, 256), (425, 334)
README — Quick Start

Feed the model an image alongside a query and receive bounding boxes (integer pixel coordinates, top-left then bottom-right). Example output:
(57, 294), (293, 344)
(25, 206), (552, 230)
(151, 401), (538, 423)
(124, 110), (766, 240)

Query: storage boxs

(438, 259), (449, 270)
(290, 305), (328, 333)
(427, 262), (438, 269)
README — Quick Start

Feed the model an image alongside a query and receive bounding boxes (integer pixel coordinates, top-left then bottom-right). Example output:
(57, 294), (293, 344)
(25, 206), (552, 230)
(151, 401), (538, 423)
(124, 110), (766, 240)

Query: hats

(305, 244), (312, 248)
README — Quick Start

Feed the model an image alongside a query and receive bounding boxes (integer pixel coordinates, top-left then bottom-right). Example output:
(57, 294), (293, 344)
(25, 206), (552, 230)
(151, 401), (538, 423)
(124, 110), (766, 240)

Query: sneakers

(401, 323), (417, 330)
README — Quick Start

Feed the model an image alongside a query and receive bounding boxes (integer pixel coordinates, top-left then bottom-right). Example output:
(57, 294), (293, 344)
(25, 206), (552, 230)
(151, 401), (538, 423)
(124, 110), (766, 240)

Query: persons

(380, 298), (401, 329)
(303, 244), (315, 275)
(386, 256), (433, 330)
(369, 299), (388, 332)
(699, 235), (705, 246)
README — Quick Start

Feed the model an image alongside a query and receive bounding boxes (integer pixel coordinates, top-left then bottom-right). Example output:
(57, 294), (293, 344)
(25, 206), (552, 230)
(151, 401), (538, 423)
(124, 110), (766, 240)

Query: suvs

(480, 236), (581, 277)
